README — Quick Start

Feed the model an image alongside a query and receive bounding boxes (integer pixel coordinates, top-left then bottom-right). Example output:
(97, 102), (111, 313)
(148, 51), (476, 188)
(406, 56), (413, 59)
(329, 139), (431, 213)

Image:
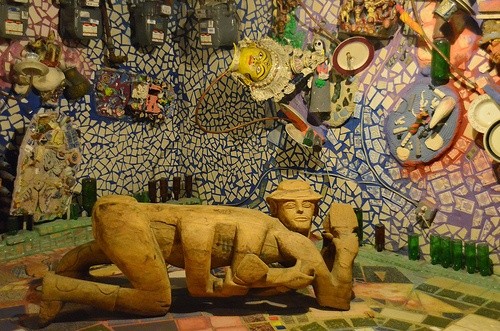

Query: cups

(452, 240), (463, 269)
(147, 180), (157, 203)
(407, 233), (420, 261)
(432, 37), (449, 83)
(375, 225), (385, 251)
(352, 208), (363, 245)
(429, 234), (440, 265)
(464, 241), (478, 272)
(477, 244), (492, 276)
(172, 177), (181, 200)
(160, 178), (168, 202)
(185, 175), (193, 198)
(439, 237), (452, 267)
(82, 178), (96, 216)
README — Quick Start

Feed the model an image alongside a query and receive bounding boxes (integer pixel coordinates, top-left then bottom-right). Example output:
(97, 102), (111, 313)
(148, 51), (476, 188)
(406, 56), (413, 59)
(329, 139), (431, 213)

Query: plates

(333, 35), (374, 75)
(483, 120), (500, 163)
(468, 94), (500, 133)
(14, 61), (49, 78)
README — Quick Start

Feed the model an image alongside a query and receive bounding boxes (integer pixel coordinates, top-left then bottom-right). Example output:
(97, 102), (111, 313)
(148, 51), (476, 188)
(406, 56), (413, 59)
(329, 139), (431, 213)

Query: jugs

(63, 65), (90, 100)
